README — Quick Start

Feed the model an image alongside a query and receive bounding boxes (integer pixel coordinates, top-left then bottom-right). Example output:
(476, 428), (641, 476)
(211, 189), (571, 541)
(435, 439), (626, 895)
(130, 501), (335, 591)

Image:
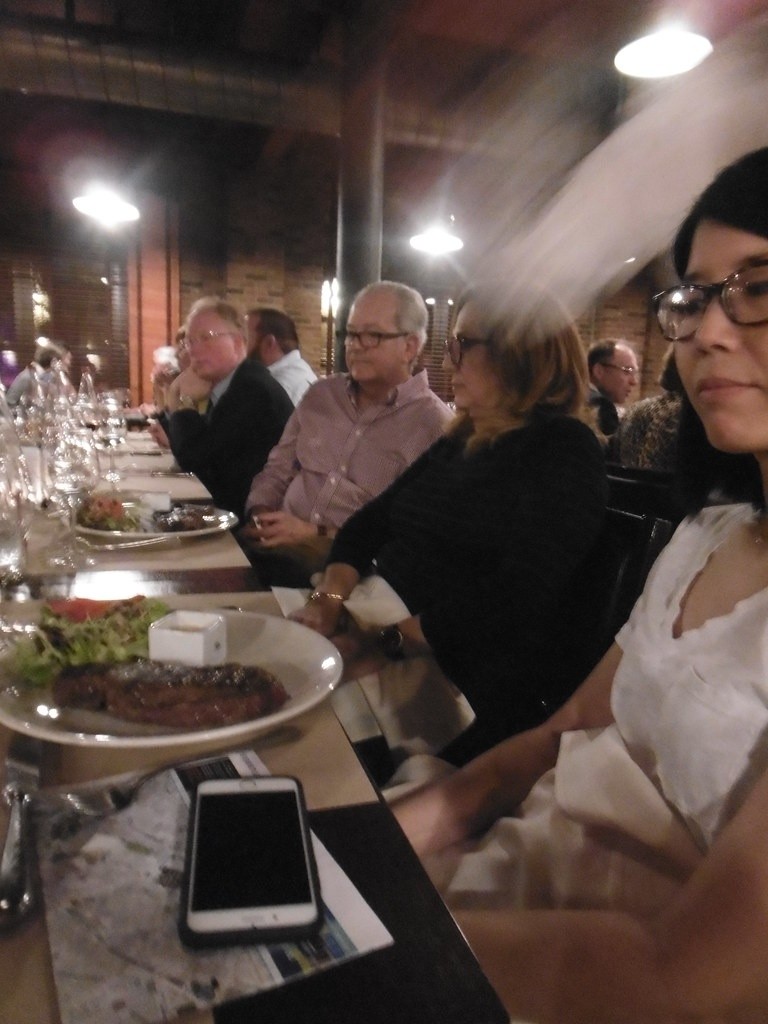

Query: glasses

(177, 330), (236, 351)
(599, 361), (638, 378)
(335, 329), (411, 349)
(444, 332), (494, 365)
(649, 256), (768, 342)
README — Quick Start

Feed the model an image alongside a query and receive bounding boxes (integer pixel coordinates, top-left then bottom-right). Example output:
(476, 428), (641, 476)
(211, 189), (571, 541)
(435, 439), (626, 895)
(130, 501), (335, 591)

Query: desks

(0, 392), (511, 1024)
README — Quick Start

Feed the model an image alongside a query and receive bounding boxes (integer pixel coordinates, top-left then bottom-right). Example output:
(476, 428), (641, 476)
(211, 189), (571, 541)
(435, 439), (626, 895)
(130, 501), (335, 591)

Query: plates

(0, 608), (344, 749)
(72, 502), (238, 538)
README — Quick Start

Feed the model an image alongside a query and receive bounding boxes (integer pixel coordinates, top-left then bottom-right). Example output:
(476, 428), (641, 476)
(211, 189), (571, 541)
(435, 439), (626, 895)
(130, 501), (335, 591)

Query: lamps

(611, 18), (713, 79)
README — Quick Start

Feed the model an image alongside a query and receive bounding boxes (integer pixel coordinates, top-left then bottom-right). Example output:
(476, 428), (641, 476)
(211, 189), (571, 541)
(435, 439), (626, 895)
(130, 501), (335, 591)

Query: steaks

(52, 653), (286, 730)
(153, 502), (215, 532)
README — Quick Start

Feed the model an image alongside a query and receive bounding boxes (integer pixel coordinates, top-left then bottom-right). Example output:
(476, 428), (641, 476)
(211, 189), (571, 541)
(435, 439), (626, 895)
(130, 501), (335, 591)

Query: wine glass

(0, 365), (127, 634)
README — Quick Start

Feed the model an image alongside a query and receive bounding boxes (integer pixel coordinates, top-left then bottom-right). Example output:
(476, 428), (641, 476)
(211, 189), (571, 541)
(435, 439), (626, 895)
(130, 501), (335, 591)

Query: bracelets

(308, 591), (343, 608)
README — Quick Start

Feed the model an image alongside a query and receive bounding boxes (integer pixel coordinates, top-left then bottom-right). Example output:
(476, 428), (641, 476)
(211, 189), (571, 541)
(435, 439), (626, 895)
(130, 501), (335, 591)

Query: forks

(56, 726), (300, 818)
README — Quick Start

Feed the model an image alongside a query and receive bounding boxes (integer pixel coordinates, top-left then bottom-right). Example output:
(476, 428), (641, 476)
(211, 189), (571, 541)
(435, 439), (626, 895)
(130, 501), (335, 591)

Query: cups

(141, 492), (170, 512)
(150, 611), (228, 668)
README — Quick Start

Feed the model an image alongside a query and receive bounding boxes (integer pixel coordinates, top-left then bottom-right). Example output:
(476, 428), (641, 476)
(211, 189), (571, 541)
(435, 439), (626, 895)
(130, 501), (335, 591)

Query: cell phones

(178, 773), (323, 949)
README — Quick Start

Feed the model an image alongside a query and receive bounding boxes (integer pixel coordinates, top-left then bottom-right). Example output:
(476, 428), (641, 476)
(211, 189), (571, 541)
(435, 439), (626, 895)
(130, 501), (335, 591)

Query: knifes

(1, 731), (46, 929)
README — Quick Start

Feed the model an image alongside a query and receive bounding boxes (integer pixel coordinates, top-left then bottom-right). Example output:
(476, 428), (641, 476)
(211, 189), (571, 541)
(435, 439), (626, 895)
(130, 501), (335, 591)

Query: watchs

(366, 622), (406, 661)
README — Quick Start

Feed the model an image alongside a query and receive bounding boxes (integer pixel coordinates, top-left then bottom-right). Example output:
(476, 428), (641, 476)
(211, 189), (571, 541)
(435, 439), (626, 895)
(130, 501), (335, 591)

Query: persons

(6, 342), (77, 406)
(389, 146), (767, 1024)
(245, 280), (457, 588)
(586, 338), (688, 471)
(288, 270), (620, 749)
(146, 297), (319, 516)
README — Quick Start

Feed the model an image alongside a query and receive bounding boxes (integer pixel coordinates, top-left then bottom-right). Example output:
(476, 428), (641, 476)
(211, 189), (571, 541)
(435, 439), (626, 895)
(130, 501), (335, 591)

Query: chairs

(534, 463), (692, 718)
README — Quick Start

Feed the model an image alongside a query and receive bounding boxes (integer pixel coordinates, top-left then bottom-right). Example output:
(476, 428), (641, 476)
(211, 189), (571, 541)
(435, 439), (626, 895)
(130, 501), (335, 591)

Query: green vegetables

(1, 593), (167, 684)
(79, 501), (144, 532)
